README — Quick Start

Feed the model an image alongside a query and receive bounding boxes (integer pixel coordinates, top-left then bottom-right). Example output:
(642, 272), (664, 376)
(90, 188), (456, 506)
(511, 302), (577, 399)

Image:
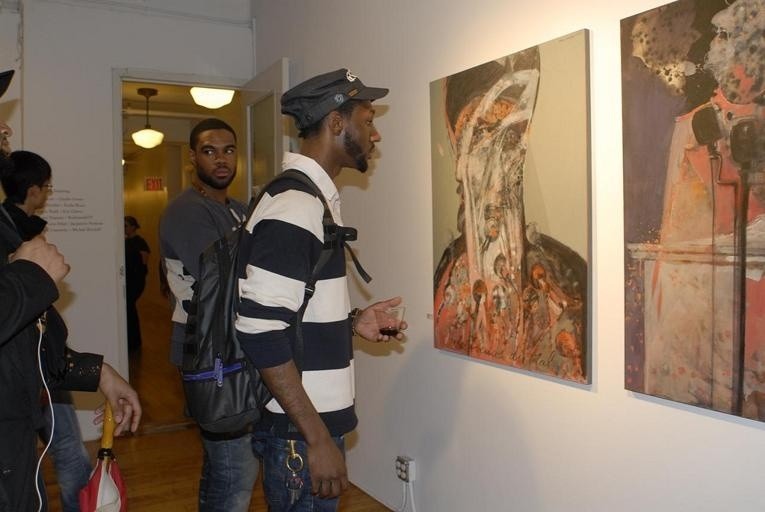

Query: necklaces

(192, 181), (208, 197)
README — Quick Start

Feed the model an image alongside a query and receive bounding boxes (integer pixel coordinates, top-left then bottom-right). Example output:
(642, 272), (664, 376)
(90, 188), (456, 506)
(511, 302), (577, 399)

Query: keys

(284, 472), (305, 504)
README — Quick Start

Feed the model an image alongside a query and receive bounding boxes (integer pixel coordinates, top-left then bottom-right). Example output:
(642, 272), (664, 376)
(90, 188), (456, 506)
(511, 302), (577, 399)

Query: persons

(124, 216), (150, 360)
(0, 68), (140, 512)
(2, 149), (96, 511)
(159, 118), (260, 512)
(235, 68), (408, 512)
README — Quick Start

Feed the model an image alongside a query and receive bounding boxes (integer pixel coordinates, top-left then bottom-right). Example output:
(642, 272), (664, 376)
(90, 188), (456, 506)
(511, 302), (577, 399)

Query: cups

(375, 308), (404, 336)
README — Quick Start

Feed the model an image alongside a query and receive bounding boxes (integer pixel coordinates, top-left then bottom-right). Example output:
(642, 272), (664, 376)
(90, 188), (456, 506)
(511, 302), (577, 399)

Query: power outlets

(393, 455), (416, 483)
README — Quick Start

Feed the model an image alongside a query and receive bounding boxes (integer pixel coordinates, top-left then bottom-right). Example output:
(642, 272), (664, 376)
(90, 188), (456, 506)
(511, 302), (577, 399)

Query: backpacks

(181, 172), (338, 436)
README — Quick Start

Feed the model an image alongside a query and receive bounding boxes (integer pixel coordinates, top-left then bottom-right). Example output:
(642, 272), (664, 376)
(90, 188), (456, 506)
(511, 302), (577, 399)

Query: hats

(279, 69), (390, 132)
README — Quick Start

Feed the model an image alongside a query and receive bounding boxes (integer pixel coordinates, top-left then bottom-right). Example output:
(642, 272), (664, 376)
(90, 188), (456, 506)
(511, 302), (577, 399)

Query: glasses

(37, 184), (54, 191)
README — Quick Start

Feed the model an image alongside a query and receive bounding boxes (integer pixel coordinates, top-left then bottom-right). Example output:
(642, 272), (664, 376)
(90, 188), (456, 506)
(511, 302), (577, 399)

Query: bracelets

(352, 308), (361, 337)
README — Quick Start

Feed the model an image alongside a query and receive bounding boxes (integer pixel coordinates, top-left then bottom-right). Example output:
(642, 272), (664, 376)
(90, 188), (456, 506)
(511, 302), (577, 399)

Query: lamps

(132, 87), (164, 150)
